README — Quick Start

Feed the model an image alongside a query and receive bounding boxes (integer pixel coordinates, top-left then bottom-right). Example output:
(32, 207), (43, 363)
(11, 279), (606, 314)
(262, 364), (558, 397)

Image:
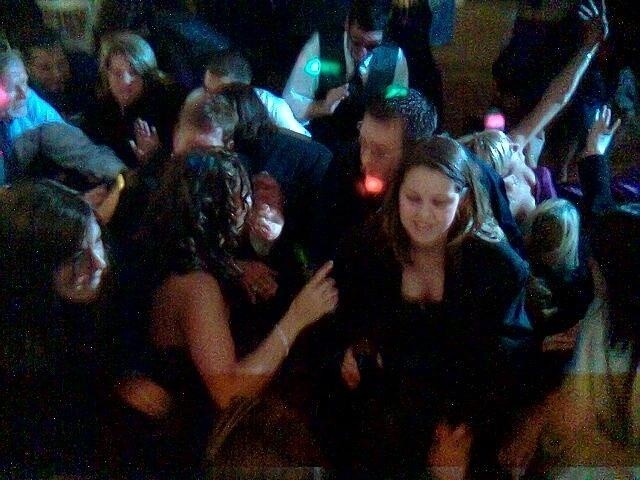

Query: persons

(0, 0), (640, 480)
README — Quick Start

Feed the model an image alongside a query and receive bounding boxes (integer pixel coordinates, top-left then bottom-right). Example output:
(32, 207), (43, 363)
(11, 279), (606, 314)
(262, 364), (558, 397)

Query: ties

(349, 64), (362, 97)
